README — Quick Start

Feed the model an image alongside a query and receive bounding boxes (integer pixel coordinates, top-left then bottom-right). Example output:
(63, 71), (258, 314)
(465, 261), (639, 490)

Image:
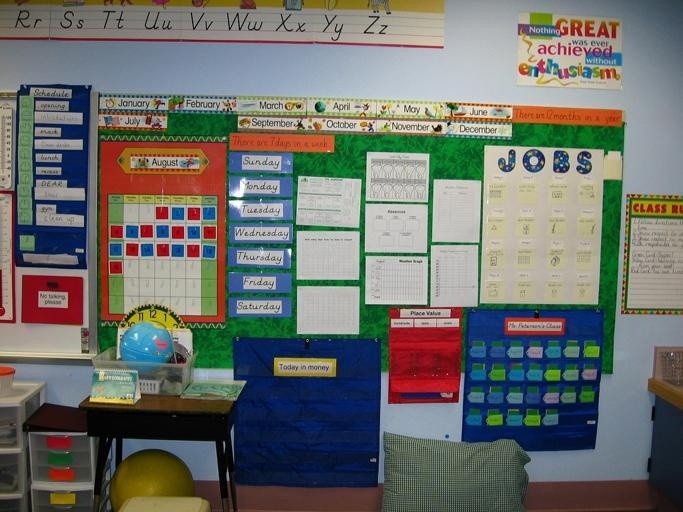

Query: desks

(78, 394), (239, 512)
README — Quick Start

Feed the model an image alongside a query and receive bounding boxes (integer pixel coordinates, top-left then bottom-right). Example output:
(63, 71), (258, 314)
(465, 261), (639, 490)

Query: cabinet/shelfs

(0, 381), (47, 511)
(28, 432), (112, 512)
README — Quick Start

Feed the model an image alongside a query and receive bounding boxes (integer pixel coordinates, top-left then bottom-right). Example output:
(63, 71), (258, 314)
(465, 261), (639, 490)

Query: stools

(118, 496), (211, 512)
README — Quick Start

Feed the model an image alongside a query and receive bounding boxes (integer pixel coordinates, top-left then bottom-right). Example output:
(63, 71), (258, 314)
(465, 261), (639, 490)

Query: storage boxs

(92, 347), (197, 396)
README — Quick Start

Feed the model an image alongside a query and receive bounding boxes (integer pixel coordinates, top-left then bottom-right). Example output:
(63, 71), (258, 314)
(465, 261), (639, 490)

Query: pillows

(381, 432), (530, 511)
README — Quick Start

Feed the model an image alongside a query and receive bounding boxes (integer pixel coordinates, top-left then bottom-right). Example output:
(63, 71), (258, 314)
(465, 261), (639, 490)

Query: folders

(22, 403), (87, 432)
